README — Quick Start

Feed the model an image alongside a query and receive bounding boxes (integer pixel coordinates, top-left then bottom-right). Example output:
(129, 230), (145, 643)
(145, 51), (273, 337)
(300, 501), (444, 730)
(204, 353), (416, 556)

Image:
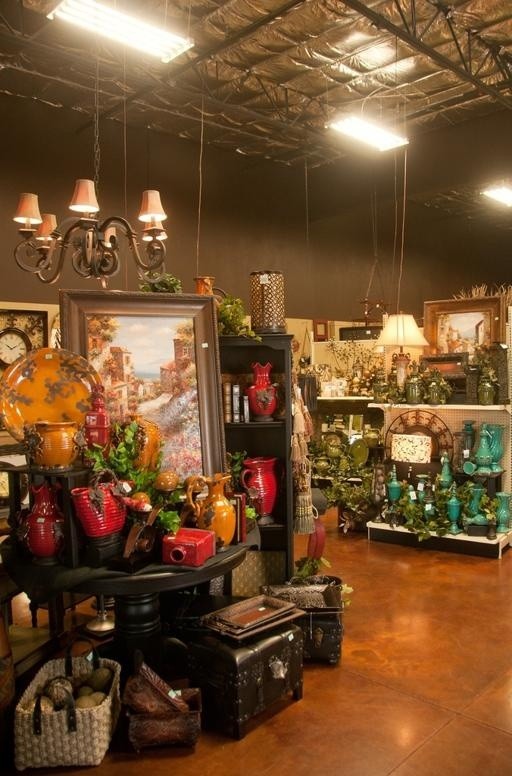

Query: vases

(491, 488), (512, 535)
(160, 526), (216, 568)
(11, 481), (67, 566)
(485, 423), (506, 474)
(69, 479), (135, 566)
(307, 440), (347, 479)
(238, 458), (282, 526)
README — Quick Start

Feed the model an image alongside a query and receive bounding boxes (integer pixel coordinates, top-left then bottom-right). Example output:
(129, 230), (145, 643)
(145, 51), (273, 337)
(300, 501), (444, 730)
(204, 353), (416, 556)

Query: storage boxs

(186, 621), (304, 740)
(125, 663), (202, 754)
(291, 616), (343, 666)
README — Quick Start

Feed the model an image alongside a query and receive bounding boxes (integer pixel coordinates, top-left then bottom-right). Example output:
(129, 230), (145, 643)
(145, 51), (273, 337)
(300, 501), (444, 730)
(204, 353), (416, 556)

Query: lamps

(391, 433), (432, 464)
(12, 34), (171, 287)
(374, 314), (430, 394)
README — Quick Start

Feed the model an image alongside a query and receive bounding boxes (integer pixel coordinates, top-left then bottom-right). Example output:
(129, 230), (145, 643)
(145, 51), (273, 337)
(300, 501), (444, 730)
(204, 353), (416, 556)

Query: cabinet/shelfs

(310, 314), (388, 482)
(365, 401), (512, 561)
(161, 333), (297, 619)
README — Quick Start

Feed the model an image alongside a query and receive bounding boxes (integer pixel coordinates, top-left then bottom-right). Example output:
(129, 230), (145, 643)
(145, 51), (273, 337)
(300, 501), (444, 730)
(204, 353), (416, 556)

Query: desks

(0, 533), (244, 689)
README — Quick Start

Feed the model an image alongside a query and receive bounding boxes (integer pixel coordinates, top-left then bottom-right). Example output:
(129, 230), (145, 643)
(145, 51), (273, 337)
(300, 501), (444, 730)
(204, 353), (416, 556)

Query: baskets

(14, 639), (122, 771)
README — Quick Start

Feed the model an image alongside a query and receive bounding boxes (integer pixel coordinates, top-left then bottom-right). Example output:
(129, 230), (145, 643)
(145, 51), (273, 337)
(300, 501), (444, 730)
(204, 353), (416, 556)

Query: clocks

(0, 309), (49, 367)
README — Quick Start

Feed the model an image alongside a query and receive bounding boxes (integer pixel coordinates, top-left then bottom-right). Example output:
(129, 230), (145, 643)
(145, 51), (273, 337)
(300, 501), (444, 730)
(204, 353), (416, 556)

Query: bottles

(386, 421), (510, 535)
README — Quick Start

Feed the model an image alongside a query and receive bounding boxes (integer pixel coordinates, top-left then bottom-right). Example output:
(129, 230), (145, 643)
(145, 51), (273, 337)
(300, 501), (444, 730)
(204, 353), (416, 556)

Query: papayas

(75, 668), (111, 714)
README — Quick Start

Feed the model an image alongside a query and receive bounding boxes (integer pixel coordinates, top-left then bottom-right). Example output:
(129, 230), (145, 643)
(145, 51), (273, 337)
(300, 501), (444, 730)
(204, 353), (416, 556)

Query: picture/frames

(59, 288), (230, 501)
(423, 296), (506, 379)
(313, 319), (328, 342)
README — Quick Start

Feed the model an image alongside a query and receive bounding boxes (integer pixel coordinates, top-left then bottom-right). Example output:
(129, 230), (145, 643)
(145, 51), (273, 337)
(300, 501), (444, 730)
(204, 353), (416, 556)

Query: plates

(198, 579), (342, 643)
(2, 346), (106, 440)
(349, 438), (369, 470)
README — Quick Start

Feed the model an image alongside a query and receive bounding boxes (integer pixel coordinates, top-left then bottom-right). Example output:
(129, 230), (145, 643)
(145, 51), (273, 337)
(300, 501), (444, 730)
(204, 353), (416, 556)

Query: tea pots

(243, 456), (279, 517)
(370, 356), (497, 405)
(312, 441), (348, 476)
(25, 479), (66, 565)
(193, 274), (229, 300)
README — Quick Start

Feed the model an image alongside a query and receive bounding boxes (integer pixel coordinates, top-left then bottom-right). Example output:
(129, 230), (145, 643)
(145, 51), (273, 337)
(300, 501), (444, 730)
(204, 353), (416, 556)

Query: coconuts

(22, 694), (54, 713)
(45, 678), (73, 710)
